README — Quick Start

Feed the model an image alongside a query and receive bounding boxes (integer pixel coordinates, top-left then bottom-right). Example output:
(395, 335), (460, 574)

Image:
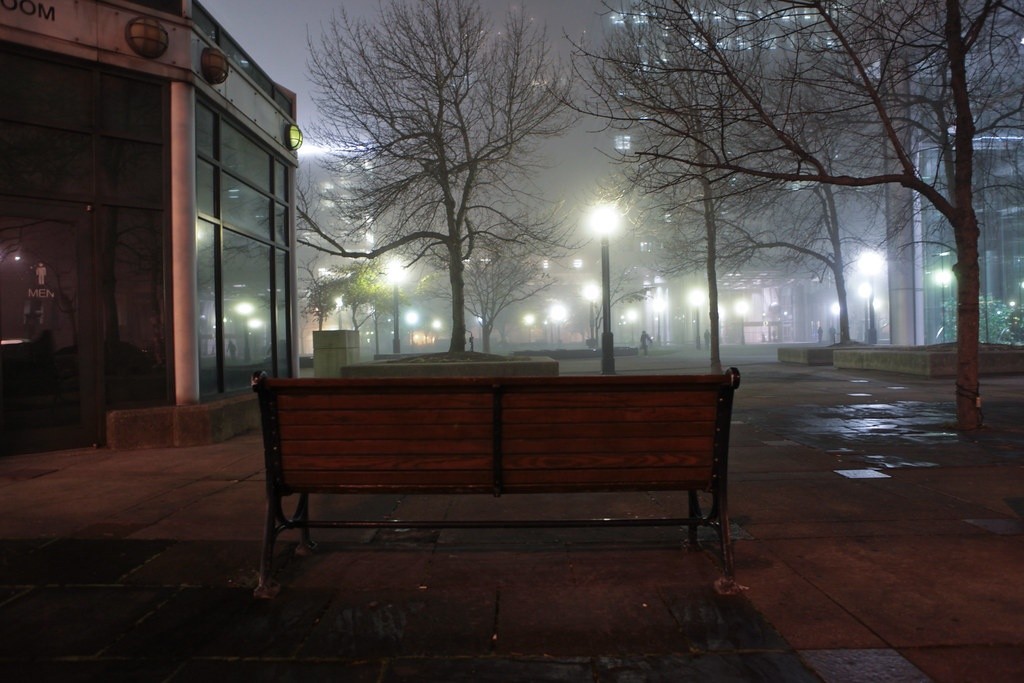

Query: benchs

(252, 368), (741, 594)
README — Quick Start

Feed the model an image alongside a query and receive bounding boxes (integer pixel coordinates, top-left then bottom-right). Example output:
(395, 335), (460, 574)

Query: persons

(818, 326), (824, 343)
(830, 326), (836, 344)
(640, 331), (653, 354)
(704, 329), (710, 348)
(227, 341), (236, 361)
